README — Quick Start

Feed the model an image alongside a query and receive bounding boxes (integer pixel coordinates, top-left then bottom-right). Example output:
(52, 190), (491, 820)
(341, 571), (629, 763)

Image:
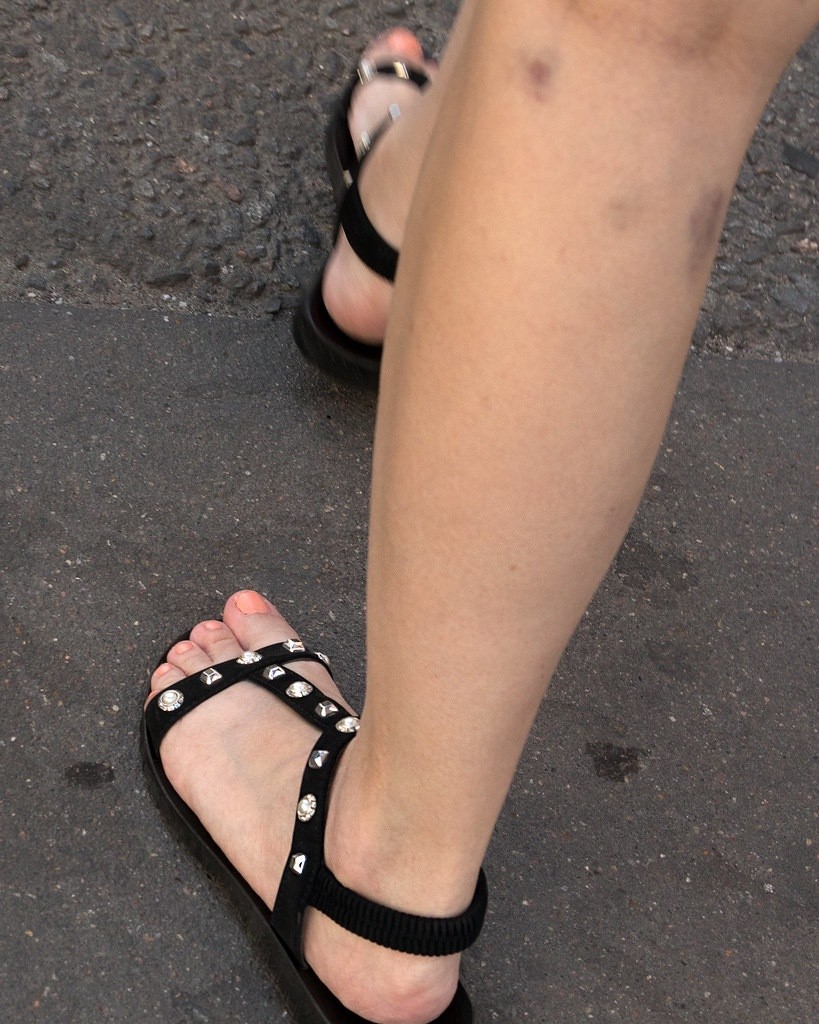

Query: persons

(142, 0), (818, 1024)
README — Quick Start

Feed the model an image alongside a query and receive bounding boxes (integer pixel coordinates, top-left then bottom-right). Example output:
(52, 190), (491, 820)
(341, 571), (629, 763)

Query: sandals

(137, 621), (489, 1024)
(287, 58), (435, 377)
